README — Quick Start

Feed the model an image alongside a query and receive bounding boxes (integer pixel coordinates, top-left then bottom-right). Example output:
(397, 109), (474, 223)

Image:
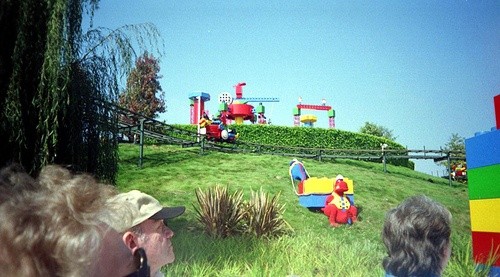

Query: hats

(119, 194), (185, 232)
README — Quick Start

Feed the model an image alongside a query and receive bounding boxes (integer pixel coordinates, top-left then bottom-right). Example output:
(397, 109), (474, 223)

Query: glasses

(125, 247), (150, 277)
